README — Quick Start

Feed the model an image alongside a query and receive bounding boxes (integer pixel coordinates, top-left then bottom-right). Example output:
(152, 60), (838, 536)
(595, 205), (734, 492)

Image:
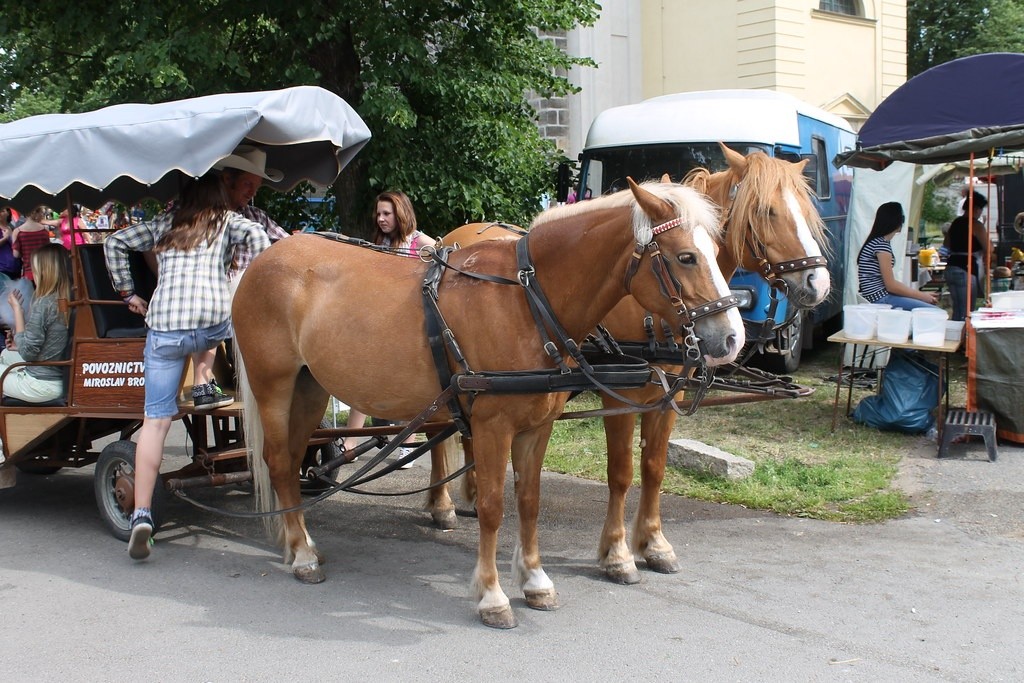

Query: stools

(938, 409), (999, 463)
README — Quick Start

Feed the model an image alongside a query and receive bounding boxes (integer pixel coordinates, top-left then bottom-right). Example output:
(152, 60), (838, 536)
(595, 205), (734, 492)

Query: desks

(919, 264), (948, 295)
(826, 327), (966, 442)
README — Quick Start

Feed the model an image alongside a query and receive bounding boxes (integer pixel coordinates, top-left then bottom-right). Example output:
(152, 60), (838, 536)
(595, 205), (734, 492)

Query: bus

(552, 86), (860, 373)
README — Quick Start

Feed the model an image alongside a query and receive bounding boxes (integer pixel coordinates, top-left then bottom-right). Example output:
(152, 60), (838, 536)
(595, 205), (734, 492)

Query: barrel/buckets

(843, 304), (949, 348)
(991, 278), (1012, 292)
(919, 250), (937, 266)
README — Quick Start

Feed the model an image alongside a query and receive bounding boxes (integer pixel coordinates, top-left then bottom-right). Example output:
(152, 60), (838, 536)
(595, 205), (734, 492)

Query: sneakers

(397, 447), (414, 468)
(191, 384), (234, 411)
(319, 447), (358, 464)
(128, 508), (154, 559)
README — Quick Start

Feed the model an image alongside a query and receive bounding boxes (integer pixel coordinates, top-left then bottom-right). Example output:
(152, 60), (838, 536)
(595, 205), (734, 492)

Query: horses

(423, 139), (832, 587)
(231, 176), (747, 630)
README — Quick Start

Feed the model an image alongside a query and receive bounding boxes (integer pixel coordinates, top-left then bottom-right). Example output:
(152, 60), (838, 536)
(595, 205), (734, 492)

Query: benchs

(0, 308), (74, 459)
(75, 243), (159, 341)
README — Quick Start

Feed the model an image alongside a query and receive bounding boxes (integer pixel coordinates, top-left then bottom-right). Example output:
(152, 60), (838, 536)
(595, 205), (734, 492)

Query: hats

(212, 145), (284, 182)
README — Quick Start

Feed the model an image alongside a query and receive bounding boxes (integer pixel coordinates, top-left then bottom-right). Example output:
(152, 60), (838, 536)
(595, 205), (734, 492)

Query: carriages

(0, 84), (839, 632)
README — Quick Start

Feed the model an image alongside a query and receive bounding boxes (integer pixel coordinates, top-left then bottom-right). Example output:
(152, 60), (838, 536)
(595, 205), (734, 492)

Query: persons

(939, 184), (995, 321)
(857, 201), (939, 310)
(372, 190), (439, 256)
(0, 144), (294, 559)
